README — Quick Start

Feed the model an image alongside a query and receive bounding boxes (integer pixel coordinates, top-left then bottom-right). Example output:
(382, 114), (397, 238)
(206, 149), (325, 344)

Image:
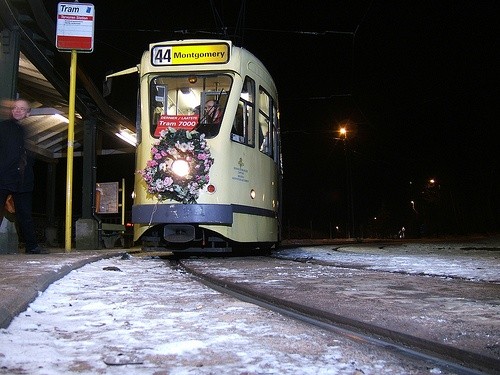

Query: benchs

(100, 223), (127, 249)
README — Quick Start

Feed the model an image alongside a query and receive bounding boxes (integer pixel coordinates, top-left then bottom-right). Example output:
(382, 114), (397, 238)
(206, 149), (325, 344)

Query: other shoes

(25, 245), (49, 254)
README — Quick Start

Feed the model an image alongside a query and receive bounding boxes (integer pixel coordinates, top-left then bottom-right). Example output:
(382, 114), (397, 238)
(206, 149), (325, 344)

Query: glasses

(204, 106), (214, 110)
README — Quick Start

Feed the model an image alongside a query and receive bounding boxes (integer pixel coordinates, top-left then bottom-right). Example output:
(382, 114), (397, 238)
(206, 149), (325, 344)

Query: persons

(204, 99), (225, 124)
(0, 99), (49, 254)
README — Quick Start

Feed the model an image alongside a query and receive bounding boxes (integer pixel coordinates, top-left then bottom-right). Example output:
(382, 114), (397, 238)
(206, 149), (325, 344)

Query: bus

(106, 38), (284, 261)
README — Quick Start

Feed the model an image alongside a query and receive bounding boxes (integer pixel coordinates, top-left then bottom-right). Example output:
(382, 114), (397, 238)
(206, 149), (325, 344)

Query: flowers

(133, 127), (214, 226)
